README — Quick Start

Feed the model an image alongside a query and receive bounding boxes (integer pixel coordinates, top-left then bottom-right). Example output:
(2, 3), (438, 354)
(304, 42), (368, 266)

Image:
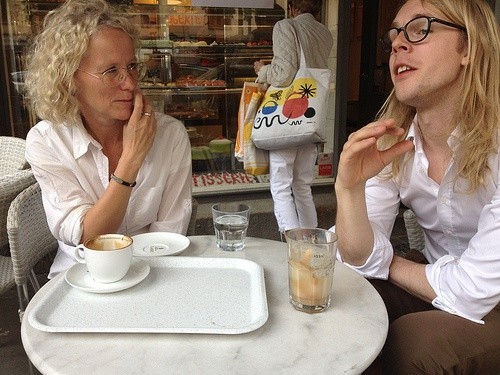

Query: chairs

(0, 135), (59, 375)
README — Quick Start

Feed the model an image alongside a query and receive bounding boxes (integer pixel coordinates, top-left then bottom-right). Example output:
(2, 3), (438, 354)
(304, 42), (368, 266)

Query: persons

(253, 0), (334, 243)
(325, 0), (500, 375)
(25, 0), (192, 278)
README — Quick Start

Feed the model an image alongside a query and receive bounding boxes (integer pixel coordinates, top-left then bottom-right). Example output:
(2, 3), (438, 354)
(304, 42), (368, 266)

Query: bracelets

(110, 174), (136, 187)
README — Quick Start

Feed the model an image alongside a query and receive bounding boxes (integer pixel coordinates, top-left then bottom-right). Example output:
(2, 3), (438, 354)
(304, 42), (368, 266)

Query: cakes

(191, 136), (231, 160)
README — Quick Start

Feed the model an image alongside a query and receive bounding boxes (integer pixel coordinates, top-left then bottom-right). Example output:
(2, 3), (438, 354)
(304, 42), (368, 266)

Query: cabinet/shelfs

(137, 45), (275, 94)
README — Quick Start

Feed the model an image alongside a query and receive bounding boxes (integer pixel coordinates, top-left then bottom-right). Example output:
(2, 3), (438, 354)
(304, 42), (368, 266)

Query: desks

(21, 234), (389, 374)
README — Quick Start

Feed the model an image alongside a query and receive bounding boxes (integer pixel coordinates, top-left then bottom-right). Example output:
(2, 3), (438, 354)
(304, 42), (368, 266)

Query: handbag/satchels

(251, 67), (331, 151)
(234, 76), (269, 175)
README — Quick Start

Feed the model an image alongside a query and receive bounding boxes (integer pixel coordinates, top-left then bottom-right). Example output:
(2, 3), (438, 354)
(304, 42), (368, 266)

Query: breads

(139, 75), (227, 87)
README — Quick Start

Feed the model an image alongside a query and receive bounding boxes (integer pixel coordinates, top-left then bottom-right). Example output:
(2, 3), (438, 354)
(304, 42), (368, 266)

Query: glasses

(77, 61), (146, 88)
(379, 15), (468, 52)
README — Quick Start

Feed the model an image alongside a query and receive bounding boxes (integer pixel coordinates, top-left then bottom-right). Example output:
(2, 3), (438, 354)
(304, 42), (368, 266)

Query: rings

(144, 113), (152, 117)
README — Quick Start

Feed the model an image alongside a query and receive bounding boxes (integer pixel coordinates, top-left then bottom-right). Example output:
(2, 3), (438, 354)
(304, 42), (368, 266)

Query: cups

(74, 234), (133, 281)
(284, 228), (338, 314)
(212, 202), (252, 251)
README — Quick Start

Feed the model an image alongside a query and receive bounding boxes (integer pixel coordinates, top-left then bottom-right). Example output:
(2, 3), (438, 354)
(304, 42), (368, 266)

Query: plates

(65, 262), (151, 293)
(129, 231), (190, 257)
